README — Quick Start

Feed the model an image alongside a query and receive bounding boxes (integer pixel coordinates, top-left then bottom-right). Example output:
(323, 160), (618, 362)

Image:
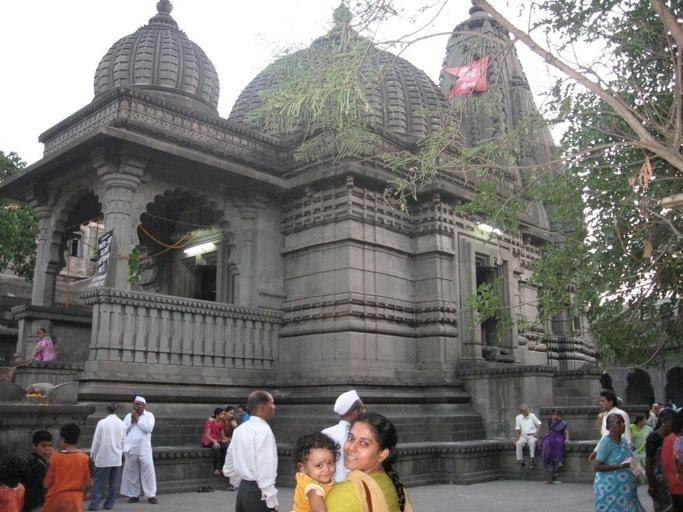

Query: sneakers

(148, 497), (158, 504)
(128, 497), (139, 503)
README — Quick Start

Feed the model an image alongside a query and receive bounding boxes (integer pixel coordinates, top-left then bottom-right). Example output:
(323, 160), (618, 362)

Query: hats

(134, 395), (147, 404)
(334, 390), (360, 416)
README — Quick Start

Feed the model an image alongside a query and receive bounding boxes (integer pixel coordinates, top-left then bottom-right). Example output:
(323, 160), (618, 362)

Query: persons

(325, 411), (413, 512)
(222, 390), (280, 512)
(119, 395), (157, 503)
(31, 327), (58, 362)
(200, 404), (250, 481)
(291, 432), (341, 512)
(588, 389), (682, 512)
(320, 390), (367, 483)
(0, 422), (93, 512)
(541, 408), (570, 479)
(515, 403), (542, 470)
(87, 402), (125, 512)
